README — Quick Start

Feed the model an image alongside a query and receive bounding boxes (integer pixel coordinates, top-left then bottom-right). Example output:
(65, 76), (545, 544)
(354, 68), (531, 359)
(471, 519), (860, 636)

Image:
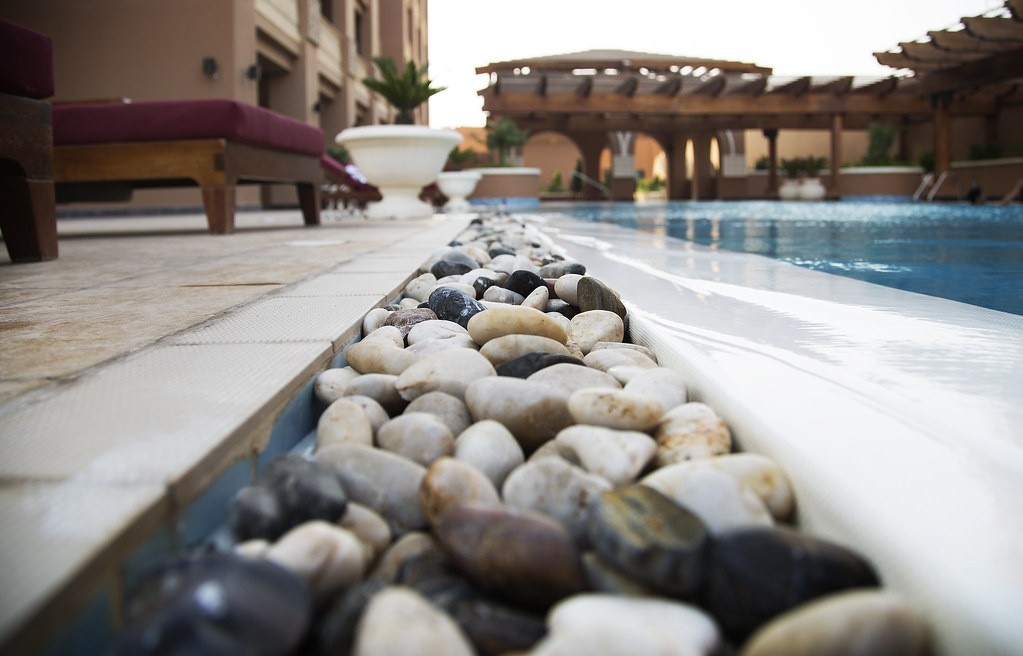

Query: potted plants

(799, 156), (828, 200)
(334, 55), (464, 219)
(778, 157), (800, 198)
(918, 151), (937, 187)
(436, 146), (481, 213)
(636, 175), (663, 199)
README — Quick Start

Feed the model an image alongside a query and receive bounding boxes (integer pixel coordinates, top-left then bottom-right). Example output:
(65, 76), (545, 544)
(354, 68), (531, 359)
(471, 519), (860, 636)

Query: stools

(0, 16), (58, 264)
(52, 99), (323, 232)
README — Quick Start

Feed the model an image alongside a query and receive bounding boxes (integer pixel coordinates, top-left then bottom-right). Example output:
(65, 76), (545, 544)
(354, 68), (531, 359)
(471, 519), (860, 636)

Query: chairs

(321, 152), (448, 211)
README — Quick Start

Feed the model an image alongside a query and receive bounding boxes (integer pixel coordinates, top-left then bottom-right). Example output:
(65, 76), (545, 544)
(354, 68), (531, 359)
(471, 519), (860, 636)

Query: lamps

(248, 66), (257, 79)
(203, 59), (216, 78)
(314, 103), (321, 111)
